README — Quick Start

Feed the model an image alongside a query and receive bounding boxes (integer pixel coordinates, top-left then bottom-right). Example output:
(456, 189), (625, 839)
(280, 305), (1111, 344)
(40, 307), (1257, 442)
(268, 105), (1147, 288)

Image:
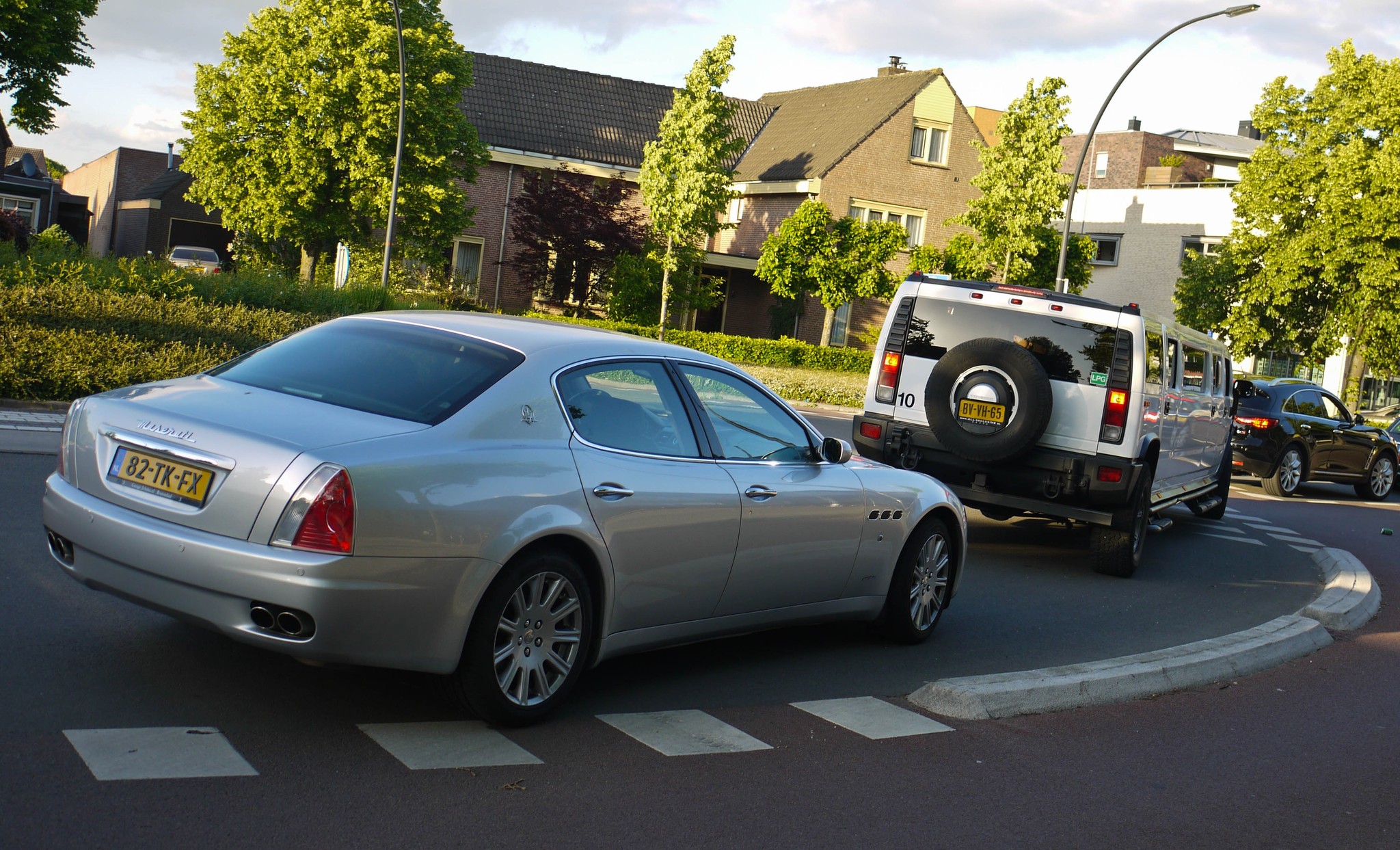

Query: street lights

(1053, 4), (1262, 294)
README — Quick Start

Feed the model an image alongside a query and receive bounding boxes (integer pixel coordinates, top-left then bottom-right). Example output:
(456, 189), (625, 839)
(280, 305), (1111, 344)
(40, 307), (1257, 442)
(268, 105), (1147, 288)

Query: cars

(161, 246), (223, 276)
(40, 309), (970, 716)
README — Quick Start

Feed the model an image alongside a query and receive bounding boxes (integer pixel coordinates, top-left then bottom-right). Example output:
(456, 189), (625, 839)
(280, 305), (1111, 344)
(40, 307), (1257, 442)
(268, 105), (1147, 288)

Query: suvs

(855, 270), (1255, 569)
(1222, 367), (1399, 501)
